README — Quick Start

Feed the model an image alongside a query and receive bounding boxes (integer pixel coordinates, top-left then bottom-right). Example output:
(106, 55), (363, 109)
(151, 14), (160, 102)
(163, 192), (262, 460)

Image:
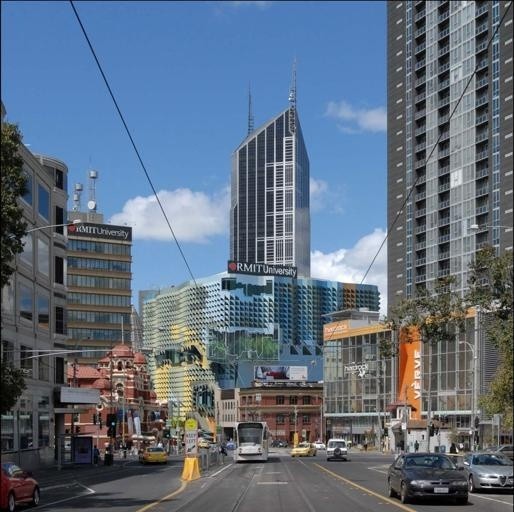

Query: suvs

(327, 438), (348, 461)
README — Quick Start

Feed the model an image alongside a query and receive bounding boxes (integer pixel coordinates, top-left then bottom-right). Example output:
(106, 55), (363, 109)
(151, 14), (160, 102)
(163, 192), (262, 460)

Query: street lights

(10, 219), (84, 238)
(298, 392), (324, 442)
(459, 340), (475, 451)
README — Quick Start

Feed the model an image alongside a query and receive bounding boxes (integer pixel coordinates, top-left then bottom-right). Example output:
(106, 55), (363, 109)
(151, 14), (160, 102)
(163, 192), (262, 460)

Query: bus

(234, 421), (269, 460)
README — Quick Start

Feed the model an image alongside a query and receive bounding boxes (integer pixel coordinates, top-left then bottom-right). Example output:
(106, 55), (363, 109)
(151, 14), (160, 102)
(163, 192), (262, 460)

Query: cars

(387, 445), (514, 504)
(226, 441), (236, 450)
(139, 447), (167, 464)
(291, 441), (326, 457)
(1, 461), (41, 512)
(198, 440), (210, 449)
(271, 438), (288, 447)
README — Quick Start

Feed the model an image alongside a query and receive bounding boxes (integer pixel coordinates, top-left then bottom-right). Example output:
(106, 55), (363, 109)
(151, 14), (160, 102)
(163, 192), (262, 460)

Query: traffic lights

(475, 415), (480, 429)
(106, 413), (117, 427)
(429, 421), (434, 437)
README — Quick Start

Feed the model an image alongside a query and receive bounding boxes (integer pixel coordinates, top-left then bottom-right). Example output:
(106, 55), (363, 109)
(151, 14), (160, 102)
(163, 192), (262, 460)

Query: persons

(364, 441), (368, 452)
(218, 445), (227, 465)
(449, 442), (456, 454)
(104, 438), (186, 459)
(93, 445), (100, 467)
(414, 440), (419, 453)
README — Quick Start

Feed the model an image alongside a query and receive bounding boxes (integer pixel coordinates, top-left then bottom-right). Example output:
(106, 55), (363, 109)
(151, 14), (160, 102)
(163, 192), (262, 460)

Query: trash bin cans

(104, 454), (113, 466)
(435, 446), (446, 454)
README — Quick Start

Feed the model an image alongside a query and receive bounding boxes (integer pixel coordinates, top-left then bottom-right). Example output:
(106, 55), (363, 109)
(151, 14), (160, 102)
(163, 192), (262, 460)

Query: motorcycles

(219, 445), (228, 456)
